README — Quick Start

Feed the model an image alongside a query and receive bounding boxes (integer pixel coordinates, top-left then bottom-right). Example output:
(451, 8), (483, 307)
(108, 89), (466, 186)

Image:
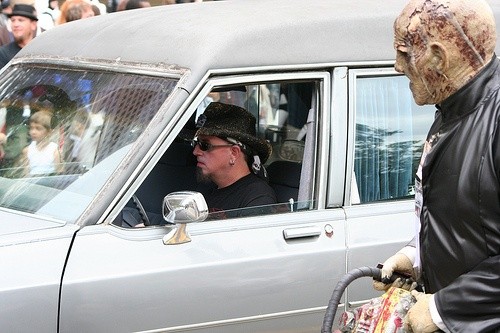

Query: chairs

(266, 160), (301, 210)
(126, 109), (217, 225)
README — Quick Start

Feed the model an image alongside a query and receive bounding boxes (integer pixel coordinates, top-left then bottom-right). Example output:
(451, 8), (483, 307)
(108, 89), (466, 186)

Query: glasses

(194, 138), (233, 151)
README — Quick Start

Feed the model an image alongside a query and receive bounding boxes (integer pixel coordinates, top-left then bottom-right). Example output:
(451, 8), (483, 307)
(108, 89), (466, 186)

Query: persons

(371, 0), (500, 333)
(0, 0), (287, 227)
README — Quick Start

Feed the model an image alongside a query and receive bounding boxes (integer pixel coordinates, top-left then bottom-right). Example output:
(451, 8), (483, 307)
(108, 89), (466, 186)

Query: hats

(7, 4), (38, 21)
(179, 102), (269, 164)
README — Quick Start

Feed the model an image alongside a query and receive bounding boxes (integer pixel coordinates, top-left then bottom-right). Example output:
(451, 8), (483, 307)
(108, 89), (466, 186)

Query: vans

(0, 0), (500, 333)
